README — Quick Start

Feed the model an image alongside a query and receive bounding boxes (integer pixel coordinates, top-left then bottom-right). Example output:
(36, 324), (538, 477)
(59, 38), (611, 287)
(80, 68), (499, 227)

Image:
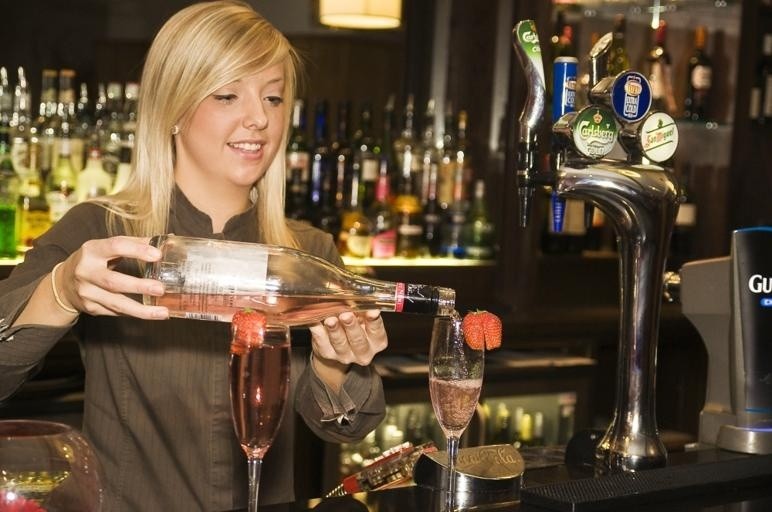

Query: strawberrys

(231, 306), (267, 349)
(461, 306), (504, 353)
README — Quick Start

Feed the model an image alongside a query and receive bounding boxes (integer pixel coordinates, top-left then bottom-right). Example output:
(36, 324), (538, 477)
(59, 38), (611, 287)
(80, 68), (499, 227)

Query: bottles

(750, 32), (771, 120)
(285, 95), (496, 261)
(677, 161), (693, 205)
(607, 12), (632, 77)
(321, 403), (546, 499)
(0, 67), (140, 259)
(645, 20), (675, 114)
(145, 235), (457, 332)
(546, 9), (575, 105)
(683, 22), (716, 119)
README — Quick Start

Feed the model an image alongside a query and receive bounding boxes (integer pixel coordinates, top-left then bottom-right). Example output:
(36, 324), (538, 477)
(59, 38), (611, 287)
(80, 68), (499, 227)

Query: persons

(0, 0), (387, 511)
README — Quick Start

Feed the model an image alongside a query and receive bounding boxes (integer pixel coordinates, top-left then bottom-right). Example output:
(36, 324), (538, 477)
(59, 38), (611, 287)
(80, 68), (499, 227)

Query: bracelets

(51, 260), (79, 316)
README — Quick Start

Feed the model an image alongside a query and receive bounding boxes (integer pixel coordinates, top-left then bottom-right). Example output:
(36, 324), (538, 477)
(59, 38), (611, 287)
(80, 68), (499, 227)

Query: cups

(1, 418), (106, 512)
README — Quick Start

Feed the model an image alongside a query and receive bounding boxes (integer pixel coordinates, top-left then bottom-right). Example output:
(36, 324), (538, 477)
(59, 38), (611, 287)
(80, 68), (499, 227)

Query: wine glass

(227, 321), (293, 510)
(428, 316), (485, 511)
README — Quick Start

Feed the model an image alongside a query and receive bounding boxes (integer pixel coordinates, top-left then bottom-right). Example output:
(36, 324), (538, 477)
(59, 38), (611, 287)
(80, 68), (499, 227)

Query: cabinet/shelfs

(317, 350), (598, 498)
(498, 1), (760, 351)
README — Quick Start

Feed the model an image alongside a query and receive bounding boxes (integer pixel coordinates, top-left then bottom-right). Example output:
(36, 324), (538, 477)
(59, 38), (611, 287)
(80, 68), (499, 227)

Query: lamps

(317, 0), (403, 31)
